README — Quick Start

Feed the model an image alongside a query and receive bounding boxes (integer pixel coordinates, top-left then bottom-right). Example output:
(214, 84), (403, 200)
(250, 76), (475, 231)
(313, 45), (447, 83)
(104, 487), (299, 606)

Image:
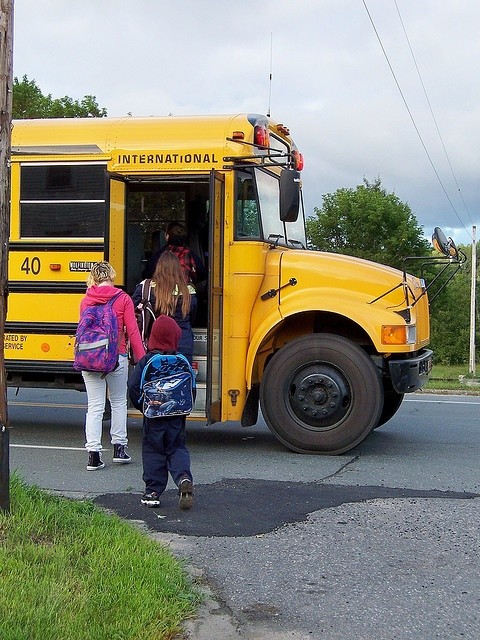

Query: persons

(145, 222), (205, 299)
(72, 260), (148, 471)
(127, 315), (197, 511)
(131, 251), (193, 369)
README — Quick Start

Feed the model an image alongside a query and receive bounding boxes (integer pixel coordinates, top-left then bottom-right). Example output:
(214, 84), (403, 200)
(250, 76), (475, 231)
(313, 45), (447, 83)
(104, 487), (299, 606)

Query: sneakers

(178, 477), (194, 511)
(87, 451), (105, 471)
(113, 444), (131, 463)
(140, 492), (161, 508)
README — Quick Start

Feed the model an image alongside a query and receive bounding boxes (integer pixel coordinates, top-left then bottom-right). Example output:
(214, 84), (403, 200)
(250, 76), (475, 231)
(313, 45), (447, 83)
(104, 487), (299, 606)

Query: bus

(3, 31), (467, 456)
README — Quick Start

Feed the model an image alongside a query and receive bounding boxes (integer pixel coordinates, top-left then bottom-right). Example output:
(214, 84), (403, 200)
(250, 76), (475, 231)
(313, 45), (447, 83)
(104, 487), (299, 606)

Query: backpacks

(73, 290), (126, 380)
(128, 279), (157, 365)
(138, 351), (196, 419)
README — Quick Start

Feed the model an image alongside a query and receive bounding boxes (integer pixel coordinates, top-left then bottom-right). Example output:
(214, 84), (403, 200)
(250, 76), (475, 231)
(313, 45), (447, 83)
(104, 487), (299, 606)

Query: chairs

(151, 229), (167, 255)
(126, 224), (145, 291)
